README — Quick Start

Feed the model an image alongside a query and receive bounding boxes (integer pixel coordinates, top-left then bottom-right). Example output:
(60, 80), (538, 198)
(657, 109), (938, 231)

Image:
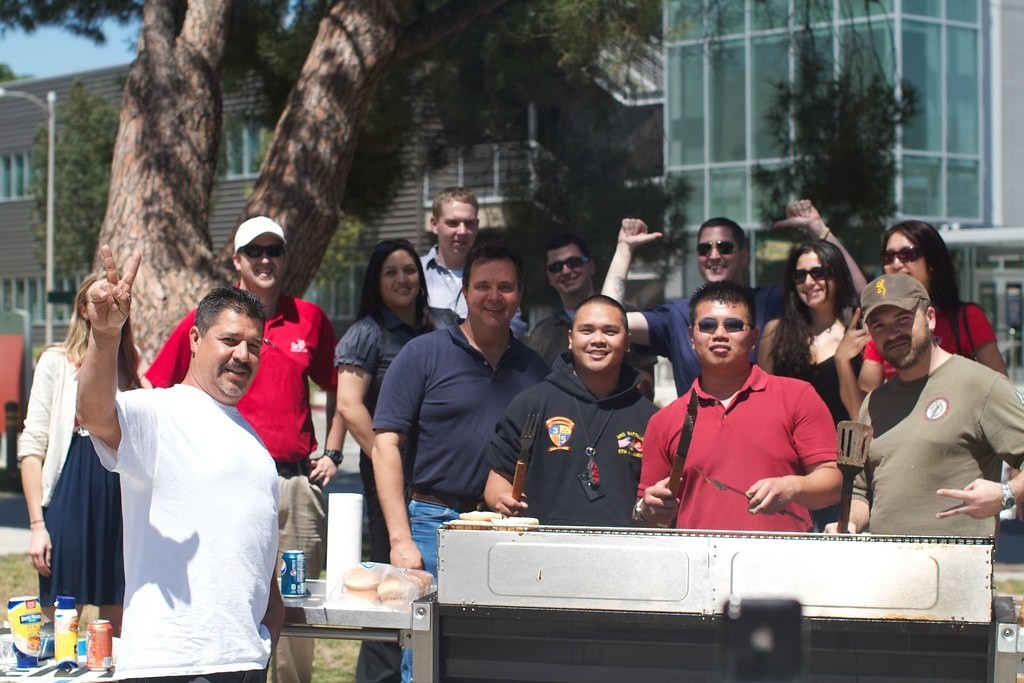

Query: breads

(343, 563), (433, 612)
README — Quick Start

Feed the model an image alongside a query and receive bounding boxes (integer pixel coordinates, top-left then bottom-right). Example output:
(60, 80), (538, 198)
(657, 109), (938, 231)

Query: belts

(408, 489), (491, 512)
(275, 460), (309, 476)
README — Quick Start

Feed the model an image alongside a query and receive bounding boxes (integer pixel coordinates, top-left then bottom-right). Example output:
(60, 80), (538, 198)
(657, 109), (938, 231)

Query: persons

(757, 239), (864, 536)
(332, 237), (463, 683)
(135, 215), (347, 683)
(632, 280), (844, 533)
(17, 268), (144, 636)
(373, 242), (553, 683)
(833, 220), (1009, 422)
(74, 240), (287, 683)
(601, 199), (867, 400)
(482, 295), (661, 528)
(525, 232), (658, 400)
(418, 188), (530, 339)
(821, 272), (1024, 535)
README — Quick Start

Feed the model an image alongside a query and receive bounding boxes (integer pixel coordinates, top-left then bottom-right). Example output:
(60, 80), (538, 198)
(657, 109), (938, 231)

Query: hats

(860, 273), (931, 324)
(234, 216), (286, 255)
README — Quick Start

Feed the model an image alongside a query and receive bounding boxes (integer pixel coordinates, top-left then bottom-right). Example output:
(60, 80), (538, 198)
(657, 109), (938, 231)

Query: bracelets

(820, 227), (830, 241)
(29, 520), (44, 524)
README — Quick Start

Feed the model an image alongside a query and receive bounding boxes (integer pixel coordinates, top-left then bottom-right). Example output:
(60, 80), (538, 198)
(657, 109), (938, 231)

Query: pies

(444, 512), (539, 531)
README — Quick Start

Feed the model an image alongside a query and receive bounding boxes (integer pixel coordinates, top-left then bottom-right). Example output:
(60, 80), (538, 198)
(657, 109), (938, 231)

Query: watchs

(1001, 482), (1015, 510)
(634, 498), (646, 523)
(323, 448), (344, 467)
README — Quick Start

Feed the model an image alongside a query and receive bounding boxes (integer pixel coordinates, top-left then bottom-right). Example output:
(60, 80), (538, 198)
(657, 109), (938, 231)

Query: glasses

(791, 267), (826, 284)
(882, 246), (921, 265)
(240, 244), (281, 257)
(697, 241), (733, 254)
(699, 318), (751, 332)
(549, 255), (587, 273)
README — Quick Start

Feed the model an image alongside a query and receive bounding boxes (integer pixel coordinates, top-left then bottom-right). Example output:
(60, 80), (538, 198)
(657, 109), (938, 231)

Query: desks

(276, 589), (1022, 683)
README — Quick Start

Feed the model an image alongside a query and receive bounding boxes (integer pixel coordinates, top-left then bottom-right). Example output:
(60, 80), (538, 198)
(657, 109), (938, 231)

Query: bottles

(7, 596), (41, 668)
(54, 597), (78, 667)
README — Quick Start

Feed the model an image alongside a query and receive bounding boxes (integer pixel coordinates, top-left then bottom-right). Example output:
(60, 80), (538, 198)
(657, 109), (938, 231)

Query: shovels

(835, 420), (874, 534)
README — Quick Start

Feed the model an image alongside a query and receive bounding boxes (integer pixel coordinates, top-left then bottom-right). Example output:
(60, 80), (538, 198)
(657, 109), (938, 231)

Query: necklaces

(576, 395), (616, 502)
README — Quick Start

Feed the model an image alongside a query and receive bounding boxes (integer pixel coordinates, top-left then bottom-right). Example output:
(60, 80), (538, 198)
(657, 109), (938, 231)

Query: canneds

(280, 549), (307, 597)
(87, 619), (113, 670)
